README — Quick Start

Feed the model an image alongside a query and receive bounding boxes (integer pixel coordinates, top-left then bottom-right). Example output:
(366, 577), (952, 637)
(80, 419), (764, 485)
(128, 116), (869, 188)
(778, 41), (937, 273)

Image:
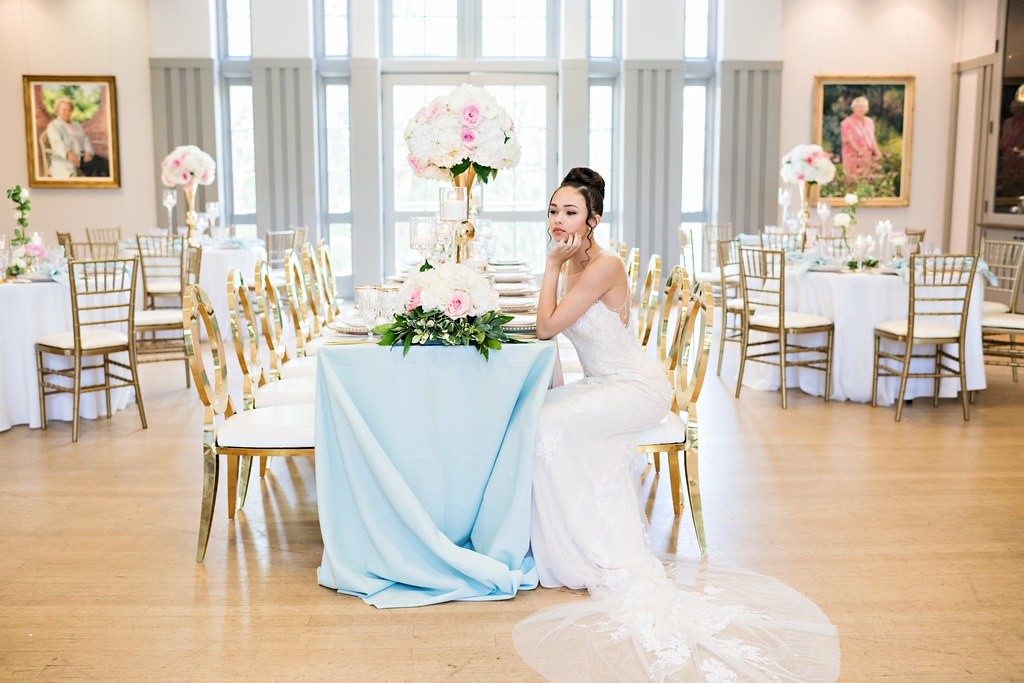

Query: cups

(768, 222), (905, 269)
(0, 234), (65, 278)
(154, 227), (188, 238)
(196, 212), (209, 232)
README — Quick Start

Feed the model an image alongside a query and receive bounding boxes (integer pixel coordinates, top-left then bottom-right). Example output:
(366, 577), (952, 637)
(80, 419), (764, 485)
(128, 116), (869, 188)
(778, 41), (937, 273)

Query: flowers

(6, 186), (49, 277)
(834, 212), (855, 259)
(844, 192), (858, 214)
(779, 141), (837, 185)
(162, 145), (215, 186)
(404, 82), (522, 184)
(369, 259), (516, 362)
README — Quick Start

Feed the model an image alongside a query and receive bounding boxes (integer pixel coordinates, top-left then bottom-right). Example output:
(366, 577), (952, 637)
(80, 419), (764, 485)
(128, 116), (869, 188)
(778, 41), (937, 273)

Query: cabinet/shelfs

(982, 227), (1024, 314)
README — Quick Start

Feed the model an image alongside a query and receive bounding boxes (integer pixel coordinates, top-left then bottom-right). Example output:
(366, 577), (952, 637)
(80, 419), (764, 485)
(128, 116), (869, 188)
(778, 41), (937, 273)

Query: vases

(837, 220), (859, 258)
(797, 181), (813, 255)
(449, 161), (476, 261)
(9, 231), (43, 274)
(837, 228), (856, 264)
(183, 184), (198, 242)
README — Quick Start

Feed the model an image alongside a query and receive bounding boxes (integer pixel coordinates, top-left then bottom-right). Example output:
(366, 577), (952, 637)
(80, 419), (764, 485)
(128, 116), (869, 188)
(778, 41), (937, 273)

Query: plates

(810, 266), (850, 272)
(326, 266), (418, 333)
(488, 258), (541, 331)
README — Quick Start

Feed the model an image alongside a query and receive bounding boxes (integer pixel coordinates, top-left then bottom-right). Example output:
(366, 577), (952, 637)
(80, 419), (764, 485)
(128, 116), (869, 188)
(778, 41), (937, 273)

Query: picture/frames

(22, 74), (121, 189)
(810, 74), (915, 208)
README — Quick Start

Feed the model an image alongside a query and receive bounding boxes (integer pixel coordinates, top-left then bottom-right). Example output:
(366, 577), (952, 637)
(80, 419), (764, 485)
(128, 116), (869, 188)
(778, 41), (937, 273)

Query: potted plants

(474, 219), (493, 241)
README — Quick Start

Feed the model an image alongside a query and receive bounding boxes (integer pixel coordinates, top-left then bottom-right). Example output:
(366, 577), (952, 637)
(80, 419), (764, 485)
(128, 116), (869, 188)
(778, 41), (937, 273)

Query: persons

(532, 167), (672, 593)
(1002, 99), (1024, 160)
(841, 97), (881, 186)
(46, 97), (93, 177)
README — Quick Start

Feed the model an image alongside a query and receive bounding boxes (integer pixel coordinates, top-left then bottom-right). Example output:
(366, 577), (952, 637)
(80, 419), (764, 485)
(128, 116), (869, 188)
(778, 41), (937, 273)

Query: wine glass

(206, 201), (218, 228)
(162, 189), (178, 247)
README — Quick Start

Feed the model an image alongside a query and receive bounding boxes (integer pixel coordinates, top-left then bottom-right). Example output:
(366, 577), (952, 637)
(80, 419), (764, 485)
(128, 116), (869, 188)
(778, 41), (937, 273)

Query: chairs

(704, 223), (734, 272)
(716, 237), (755, 376)
(677, 227), (739, 330)
(971, 313), (1024, 404)
(814, 237), (857, 258)
(644, 265), (690, 474)
(626, 246), (640, 302)
(758, 229), (807, 253)
(735, 246), (835, 407)
(630, 280), (715, 559)
(35, 226), (366, 562)
(615, 240), (627, 264)
(904, 228), (926, 254)
(983, 228), (1024, 381)
(872, 248), (983, 422)
(635, 254), (664, 352)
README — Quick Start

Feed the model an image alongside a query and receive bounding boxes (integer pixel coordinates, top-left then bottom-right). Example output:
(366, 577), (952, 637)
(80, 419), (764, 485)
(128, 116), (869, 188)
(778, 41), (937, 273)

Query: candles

(410, 217), (437, 249)
(468, 248), (488, 268)
(888, 230), (907, 246)
(439, 187), (468, 221)
(434, 221), (451, 240)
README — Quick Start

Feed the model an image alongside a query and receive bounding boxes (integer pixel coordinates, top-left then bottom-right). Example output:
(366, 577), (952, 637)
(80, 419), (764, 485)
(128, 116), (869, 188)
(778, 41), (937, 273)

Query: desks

(117, 233), (268, 344)
(316, 254), (564, 610)
(732, 252), (998, 408)
(0, 259), (138, 434)
